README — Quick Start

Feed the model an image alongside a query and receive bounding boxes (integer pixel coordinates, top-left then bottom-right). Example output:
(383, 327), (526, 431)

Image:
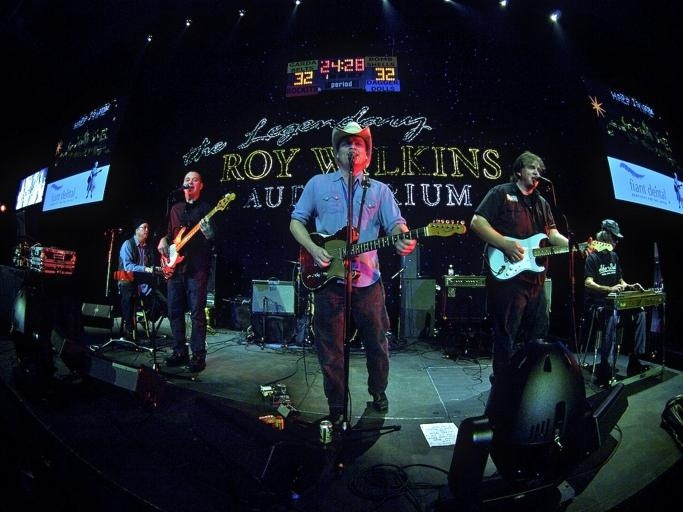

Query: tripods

(300, 166), (401, 481)
(245, 297), (285, 349)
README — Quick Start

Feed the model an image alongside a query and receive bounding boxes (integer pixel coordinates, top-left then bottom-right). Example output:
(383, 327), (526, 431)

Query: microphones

(534, 175), (553, 185)
(172, 184), (188, 195)
(274, 301), (279, 314)
(348, 152), (354, 160)
(104, 228), (124, 236)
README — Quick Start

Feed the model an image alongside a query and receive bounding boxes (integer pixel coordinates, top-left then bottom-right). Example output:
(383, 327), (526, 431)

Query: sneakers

(185, 359), (206, 372)
(163, 352), (190, 366)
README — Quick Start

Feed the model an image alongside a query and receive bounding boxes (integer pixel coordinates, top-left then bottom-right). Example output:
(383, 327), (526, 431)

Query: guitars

(161, 192), (236, 278)
(484, 231), (613, 281)
(299, 220), (466, 290)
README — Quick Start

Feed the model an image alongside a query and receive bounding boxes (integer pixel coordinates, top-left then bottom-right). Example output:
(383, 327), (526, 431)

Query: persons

(84, 160), (99, 199)
(111, 216), (170, 340)
(286, 120), (417, 428)
(577, 218), (654, 385)
(469, 149), (597, 389)
(153, 168), (219, 374)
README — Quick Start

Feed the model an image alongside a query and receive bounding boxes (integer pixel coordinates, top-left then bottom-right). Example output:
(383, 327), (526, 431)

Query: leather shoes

(627, 362), (652, 377)
(367, 386), (388, 412)
(328, 408), (346, 429)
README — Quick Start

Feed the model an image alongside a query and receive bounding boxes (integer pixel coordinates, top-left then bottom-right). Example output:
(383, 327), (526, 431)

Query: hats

(331, 121), (373, 168)
(602, 219), (624, 238)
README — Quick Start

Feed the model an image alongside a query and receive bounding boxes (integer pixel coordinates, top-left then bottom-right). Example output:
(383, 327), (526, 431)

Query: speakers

(173, 395), (309, 512)
(81, 303), (114, 328)
(251, 280), (296, 315)
(83, 353), (165, 412)
(439, 275), (488, 319)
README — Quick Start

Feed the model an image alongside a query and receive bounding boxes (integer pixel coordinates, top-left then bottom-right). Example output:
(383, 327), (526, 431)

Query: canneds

(320, 421), (333, 445)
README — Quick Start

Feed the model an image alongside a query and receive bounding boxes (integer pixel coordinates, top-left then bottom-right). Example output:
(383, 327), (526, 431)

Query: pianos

(115, 270), (165, 286)
(575, 289), (667, 311)
